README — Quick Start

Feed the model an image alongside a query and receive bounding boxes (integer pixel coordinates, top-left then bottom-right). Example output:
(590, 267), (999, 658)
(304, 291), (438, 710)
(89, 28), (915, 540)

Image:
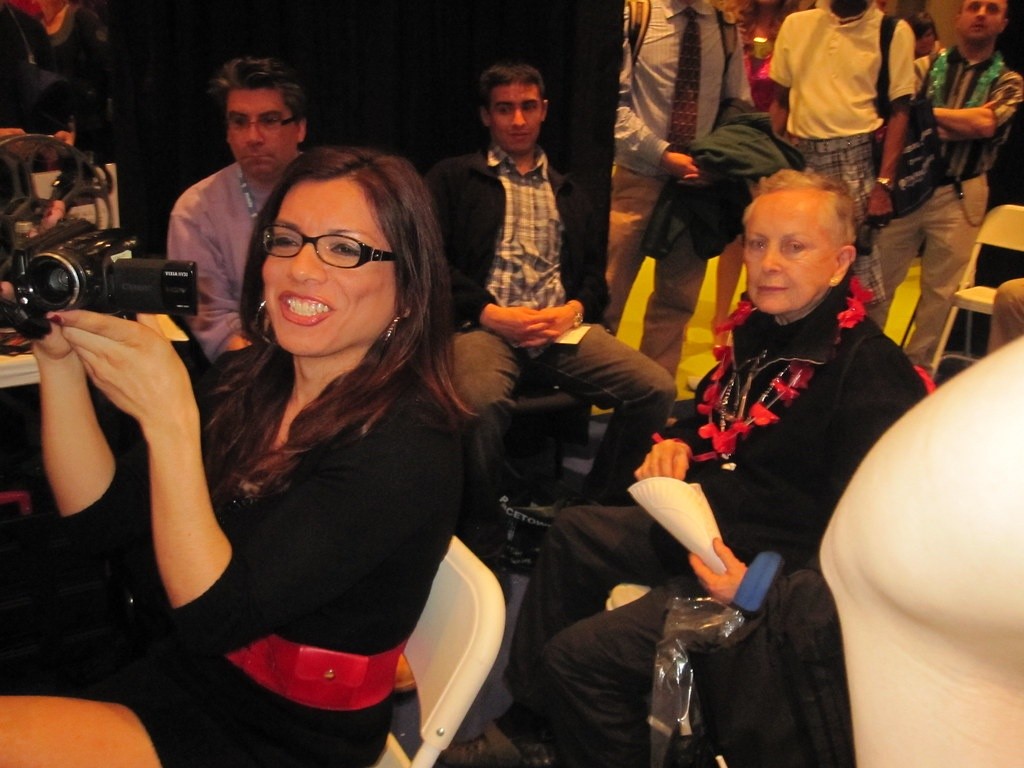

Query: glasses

(225, 116), (299, 131)
(262, 222), (397, 269)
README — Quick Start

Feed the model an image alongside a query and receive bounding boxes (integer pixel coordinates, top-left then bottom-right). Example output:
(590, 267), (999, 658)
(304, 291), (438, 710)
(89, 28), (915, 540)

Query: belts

(792, 132), (870, 156)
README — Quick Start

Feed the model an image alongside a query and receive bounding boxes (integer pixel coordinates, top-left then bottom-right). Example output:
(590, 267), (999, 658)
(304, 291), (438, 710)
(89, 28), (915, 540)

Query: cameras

(14, 219), (199, 318)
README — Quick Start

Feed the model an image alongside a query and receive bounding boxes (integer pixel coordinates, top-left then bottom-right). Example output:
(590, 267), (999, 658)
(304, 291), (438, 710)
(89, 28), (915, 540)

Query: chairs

(932, 205), (1024, 381)
(511, 382), (589, 482)
(373, 535), (508, 768)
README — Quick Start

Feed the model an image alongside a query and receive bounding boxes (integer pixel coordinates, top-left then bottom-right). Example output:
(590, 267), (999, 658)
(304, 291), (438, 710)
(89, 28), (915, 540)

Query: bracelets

(877, 177), (894, 191)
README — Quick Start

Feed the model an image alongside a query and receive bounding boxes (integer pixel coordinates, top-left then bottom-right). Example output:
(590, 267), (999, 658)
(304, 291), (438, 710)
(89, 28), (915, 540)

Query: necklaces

(697, 275), (874, 455)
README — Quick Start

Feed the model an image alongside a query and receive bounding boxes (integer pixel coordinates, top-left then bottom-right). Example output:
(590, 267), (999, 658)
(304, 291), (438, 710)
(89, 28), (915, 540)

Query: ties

(668, 9), (702, 153)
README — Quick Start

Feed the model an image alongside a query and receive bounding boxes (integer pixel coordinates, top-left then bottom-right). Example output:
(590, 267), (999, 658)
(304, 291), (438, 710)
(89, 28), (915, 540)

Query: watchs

(568, 304), (583, 328)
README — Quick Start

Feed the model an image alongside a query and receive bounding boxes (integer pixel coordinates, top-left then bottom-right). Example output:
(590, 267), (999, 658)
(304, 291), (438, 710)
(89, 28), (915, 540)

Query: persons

(1, 0), (123, 168)
(602, 0), (1024, 381)
(166, 57), (308, 362)
(420, 62), (676, 508)
(441, 169), (927, 768)
(0, 146), (464, 768)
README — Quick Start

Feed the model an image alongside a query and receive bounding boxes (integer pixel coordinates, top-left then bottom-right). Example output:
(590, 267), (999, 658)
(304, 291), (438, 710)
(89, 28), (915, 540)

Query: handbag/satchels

(872, 15), (943, 219)
(501, 456), (596, 571)
